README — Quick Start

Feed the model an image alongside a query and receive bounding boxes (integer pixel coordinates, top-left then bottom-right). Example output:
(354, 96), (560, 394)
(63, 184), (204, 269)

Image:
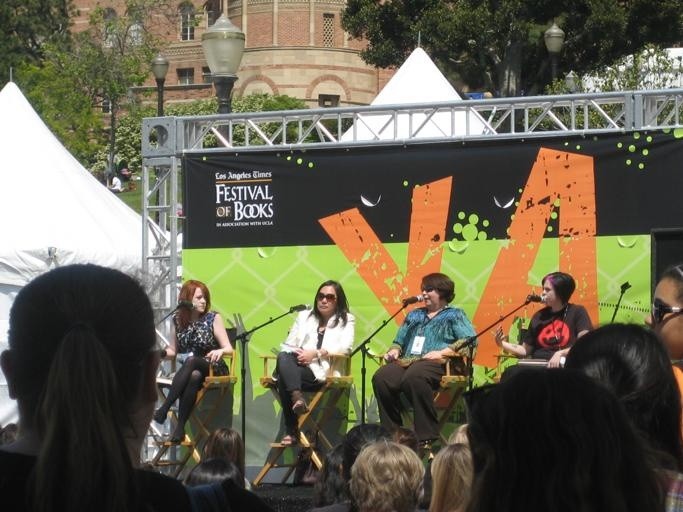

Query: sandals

(281, 400), (308, 446)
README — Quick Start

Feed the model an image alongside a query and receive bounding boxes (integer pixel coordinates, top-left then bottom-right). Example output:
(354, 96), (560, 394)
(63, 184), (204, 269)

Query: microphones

(179, 300), (197, 310)
(529, 295), (544, 302)
(403, 295), (423, 304)
(293, 304), (312, 311)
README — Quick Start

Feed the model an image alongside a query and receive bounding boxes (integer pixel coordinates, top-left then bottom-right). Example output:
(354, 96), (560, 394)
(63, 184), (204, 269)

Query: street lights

(201, 11), (244, 146)
(151, 53), (170, 226)
(543, 19), (566, 81)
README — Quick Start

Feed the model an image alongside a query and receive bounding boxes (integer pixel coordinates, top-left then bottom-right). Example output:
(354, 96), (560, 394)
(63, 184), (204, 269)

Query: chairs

(251, 328), (353, 491)
(150, 328), (237, 480)
(377, 334), (476, 458)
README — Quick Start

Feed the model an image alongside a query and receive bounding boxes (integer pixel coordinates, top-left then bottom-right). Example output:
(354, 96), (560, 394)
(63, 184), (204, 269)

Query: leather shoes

(418, 439), (435, 447)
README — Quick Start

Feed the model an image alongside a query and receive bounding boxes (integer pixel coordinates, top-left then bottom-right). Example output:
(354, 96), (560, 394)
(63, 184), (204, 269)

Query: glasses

(422, 285), (439, 293)
(652, 299), (683, 326)
(316, 292), (337, 303)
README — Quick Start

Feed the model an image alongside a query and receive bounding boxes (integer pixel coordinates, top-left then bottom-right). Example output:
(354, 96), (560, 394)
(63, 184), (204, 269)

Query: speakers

(651, 227), (683, 304)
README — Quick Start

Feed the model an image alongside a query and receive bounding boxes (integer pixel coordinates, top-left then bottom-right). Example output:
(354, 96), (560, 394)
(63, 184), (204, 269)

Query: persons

(0, 264), (183, 512)
(154, 280), (234, 442)
(186, 262), (682, 511)
